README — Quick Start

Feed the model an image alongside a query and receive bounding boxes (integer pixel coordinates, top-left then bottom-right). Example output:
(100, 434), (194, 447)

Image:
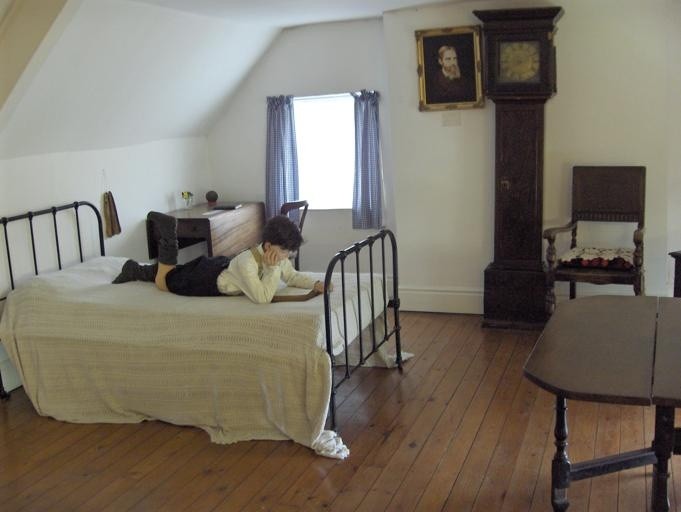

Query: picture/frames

(412, 22), (486, 110)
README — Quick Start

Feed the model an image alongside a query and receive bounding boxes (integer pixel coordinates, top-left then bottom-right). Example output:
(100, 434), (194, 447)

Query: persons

(427, 45), (476, 104)
(111, 210), (335, 306)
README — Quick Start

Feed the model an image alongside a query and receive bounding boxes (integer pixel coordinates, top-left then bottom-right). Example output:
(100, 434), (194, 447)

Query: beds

(0, 193), (411, 459)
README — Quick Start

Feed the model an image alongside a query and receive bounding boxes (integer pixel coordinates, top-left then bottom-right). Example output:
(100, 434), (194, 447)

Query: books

(270, 284), (322, 303)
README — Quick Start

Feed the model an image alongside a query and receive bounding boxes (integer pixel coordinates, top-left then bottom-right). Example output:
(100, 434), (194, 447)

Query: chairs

(279, 198), (307, 272)
(542, 165), (645, 324)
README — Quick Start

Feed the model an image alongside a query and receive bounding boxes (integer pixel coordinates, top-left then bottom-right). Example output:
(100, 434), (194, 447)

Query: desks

(147, 201), (267, 260)
(521, 294), (681, 512)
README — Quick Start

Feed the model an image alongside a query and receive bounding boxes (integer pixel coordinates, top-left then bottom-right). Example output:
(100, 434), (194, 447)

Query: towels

(103, 191), (121, 237)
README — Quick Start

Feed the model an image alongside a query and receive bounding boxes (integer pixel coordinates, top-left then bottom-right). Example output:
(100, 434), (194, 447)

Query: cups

(184, 197), (192, 209)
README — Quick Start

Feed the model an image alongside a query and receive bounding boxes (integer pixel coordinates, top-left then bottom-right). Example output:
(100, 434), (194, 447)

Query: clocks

(470, 3), (564, 332)
